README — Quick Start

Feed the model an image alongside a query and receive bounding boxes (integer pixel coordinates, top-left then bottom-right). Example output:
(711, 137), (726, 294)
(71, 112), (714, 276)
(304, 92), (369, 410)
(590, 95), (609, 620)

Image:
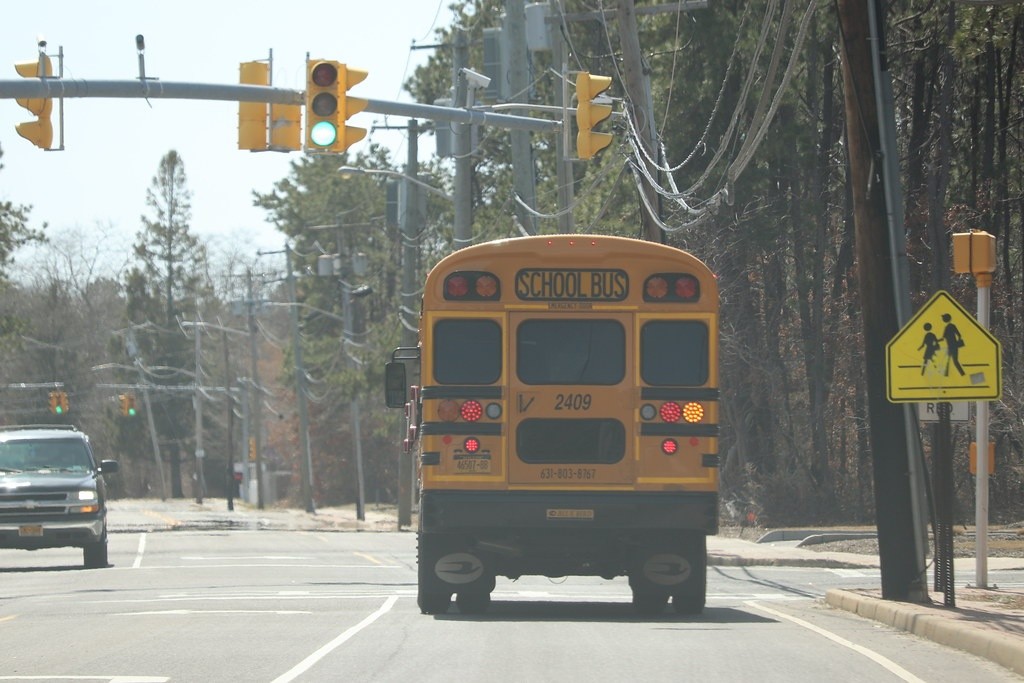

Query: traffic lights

(576, 68), (613, 161)
(14, 53), (52, 150)
(305, 59), (344, 157)
(47, 392), (56, 415)
(126, 396), (135, 416)
(248, 437), (256, 463)
(340, 62), (369, 151)
(54, 392), (63, 415)
(118, 394), (127, 416)
(62, 392), (69, 413)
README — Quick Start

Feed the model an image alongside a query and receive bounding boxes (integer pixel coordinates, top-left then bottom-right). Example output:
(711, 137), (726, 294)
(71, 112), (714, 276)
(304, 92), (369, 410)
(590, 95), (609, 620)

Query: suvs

(0, 424), (119, 567)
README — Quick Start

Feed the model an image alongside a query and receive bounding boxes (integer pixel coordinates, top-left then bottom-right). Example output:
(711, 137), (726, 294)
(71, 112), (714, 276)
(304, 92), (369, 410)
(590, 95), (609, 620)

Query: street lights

(178, 321), (266, 511)
(261, 300), (364, 523)
(88, 362), (169, 501)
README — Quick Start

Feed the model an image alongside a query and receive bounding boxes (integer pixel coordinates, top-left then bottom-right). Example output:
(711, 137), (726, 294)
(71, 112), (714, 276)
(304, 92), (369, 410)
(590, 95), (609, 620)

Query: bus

(383, 234), (722, 616)
